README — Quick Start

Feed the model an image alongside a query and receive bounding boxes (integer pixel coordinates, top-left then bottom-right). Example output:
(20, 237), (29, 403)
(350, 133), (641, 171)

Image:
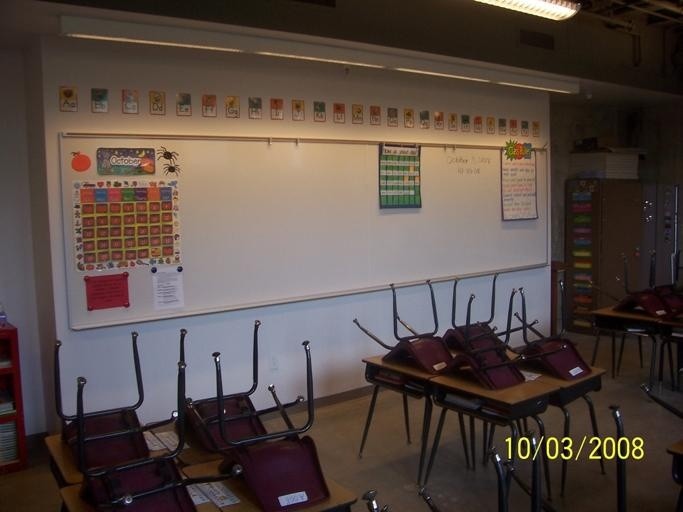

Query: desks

(519, 363), (607, 499)
(471, 350), (520, 472)
(421, 374), (559, 506)
(657, 320), (683, 394)
(147, 416), (261, 481)
(194, 465), (357, 511)
(358, 352), (457, 488)
(44, 420), (178, 490)
(58, 467), (221, 511)
(591, 306), (660, 390)
(611, 407), (683, 511)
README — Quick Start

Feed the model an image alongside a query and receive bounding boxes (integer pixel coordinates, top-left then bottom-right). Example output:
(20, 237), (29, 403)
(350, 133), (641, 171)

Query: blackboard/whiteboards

(57, 127), (549, 333)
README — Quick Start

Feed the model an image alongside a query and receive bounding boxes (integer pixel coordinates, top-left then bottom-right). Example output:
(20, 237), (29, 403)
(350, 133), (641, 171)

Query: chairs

(55, 332), (179, 475)
(353, 280), (453, 375)
(452, 288), (525, 390)
(442, 272), (539, 369)
(175, 320), (304, 455)
(622, 248), (683, 319)
(212, 341), (331, 511)
(593, 250), (671, 318)
(79, 363), (244, 511)
(519, 280), (592, 381)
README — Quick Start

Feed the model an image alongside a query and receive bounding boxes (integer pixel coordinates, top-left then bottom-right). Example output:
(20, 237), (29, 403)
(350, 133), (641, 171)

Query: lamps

(476, 1), (580, 23)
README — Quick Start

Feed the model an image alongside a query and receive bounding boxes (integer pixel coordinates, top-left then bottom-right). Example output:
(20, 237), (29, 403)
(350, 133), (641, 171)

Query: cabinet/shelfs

(0, 323), (28, 477)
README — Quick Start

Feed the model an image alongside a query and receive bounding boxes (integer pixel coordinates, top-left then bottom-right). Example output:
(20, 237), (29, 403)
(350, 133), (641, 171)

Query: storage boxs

(569, 153), (639, 179)
